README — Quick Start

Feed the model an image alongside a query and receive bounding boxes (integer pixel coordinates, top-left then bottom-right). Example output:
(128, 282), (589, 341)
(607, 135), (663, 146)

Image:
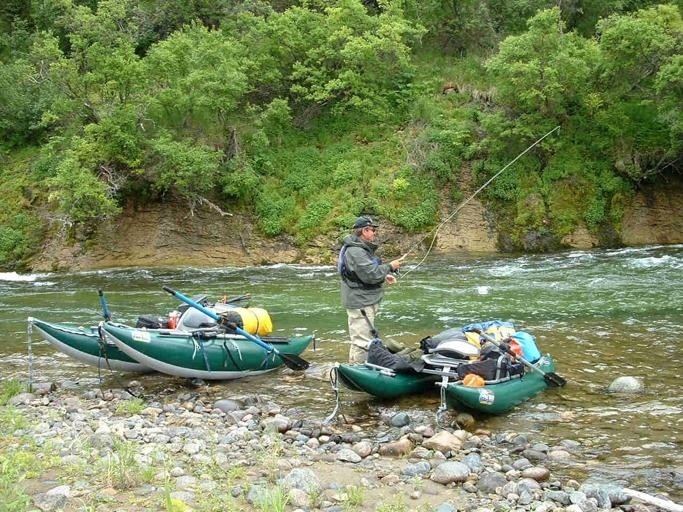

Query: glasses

(368, 229), (376, 232)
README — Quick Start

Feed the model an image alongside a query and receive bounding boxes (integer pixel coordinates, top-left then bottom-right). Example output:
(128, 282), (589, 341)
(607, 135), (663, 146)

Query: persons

(336, 214), (400, 364)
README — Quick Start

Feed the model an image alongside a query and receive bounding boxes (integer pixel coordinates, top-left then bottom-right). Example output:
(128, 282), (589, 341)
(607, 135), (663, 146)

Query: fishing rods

(389, 125), (560, 279)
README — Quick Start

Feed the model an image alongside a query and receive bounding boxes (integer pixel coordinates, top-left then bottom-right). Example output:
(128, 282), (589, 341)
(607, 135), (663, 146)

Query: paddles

(159, 331), (288, 345)
(471, 328), (567, 389)
(162, 286), (309, 371)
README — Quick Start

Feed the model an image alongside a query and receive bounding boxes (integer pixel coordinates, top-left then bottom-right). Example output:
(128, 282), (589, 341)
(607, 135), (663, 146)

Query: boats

(334, 354), (553, 414)
(28, 316), (315, 379)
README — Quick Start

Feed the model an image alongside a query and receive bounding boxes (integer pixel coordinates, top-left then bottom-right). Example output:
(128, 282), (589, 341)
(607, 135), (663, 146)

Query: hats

(352, 215), (380, 229)
